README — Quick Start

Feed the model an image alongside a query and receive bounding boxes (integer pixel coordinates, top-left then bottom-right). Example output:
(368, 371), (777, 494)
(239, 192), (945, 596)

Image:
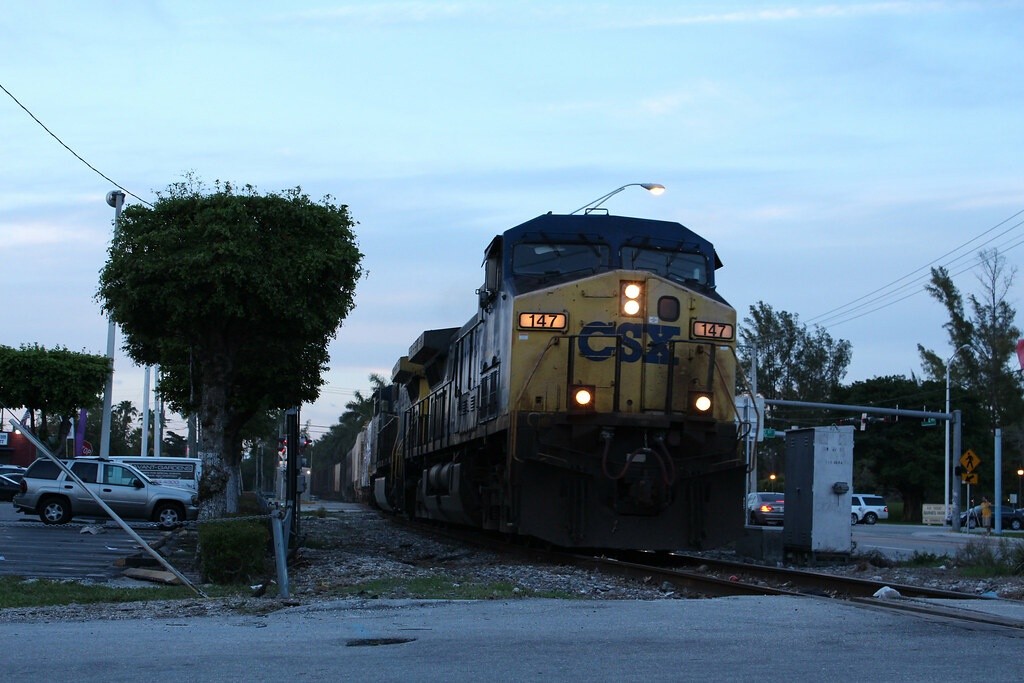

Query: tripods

(959, 499), (983, 536)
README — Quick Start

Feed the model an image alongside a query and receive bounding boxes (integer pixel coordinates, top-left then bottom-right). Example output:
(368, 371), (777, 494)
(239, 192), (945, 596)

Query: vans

(850, 491), (889, 525)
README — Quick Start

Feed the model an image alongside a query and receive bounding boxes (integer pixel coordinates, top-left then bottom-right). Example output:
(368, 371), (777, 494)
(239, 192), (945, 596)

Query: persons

(981, 496), (992, 536)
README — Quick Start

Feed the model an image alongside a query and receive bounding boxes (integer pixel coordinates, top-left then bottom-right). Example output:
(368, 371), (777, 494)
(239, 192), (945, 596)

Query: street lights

(945, 343), (973, 522)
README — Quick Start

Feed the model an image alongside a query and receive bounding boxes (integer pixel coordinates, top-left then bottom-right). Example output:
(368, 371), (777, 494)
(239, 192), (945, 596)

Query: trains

(317, 199), (760, 552)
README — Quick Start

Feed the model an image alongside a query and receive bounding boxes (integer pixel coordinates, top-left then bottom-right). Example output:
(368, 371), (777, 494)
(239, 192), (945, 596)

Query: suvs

(12, 455), (201, 532)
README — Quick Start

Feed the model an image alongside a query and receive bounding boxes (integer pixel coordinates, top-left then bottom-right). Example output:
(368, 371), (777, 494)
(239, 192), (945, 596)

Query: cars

(746, 491), (786, 524)
(946, 503), (1024, 532)
(0, 464), (29, 499)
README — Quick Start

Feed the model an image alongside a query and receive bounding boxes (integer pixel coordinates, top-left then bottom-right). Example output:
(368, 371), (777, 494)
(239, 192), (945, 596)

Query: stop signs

(82, 441), (94, 456)
